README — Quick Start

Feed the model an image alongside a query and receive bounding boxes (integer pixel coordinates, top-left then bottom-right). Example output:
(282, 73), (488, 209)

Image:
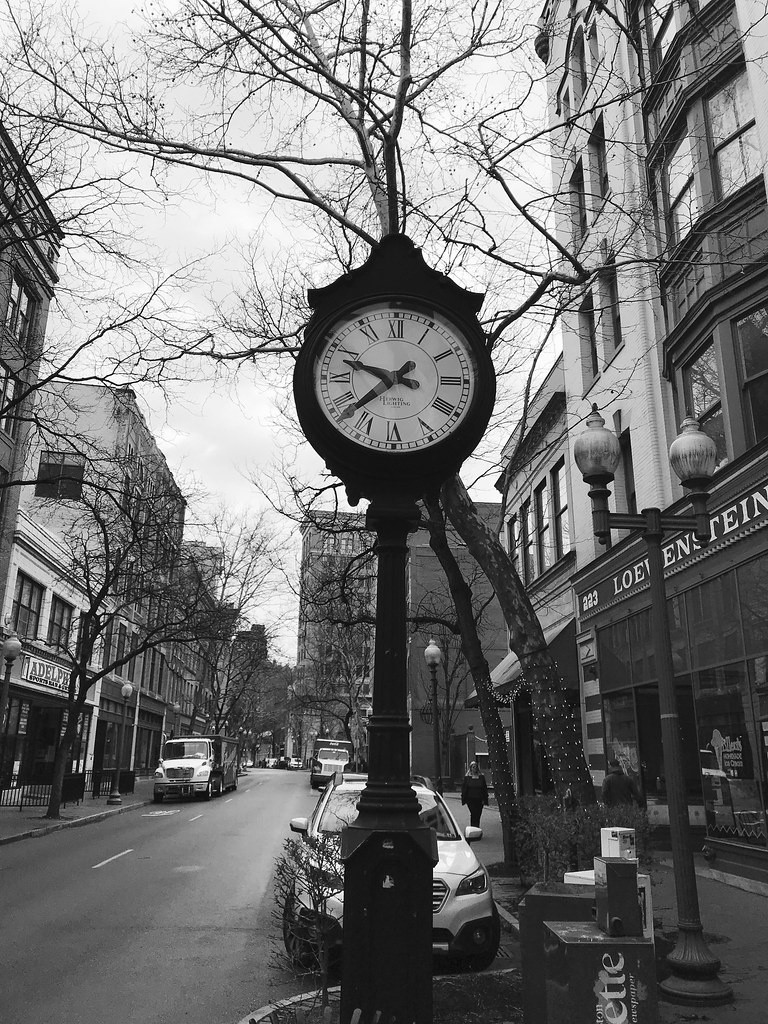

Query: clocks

(293, 234), (497, 533)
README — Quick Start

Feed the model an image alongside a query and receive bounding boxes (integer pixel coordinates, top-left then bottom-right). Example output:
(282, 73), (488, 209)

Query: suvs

(283, 772), (501, 973)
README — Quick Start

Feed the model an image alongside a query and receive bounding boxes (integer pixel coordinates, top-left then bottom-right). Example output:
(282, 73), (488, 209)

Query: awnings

(466, 619), (578, 707)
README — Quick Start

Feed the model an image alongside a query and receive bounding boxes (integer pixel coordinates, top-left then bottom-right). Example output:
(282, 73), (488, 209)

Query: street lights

(225, 721), (228, 736)
(425, 639), (443, 799)
(0, 632), (21, 731)
(574, 402), (734, 1008)
(326, 728), (329, 739)
(169, 702), (180, 740)
(107, 681), (132, 805)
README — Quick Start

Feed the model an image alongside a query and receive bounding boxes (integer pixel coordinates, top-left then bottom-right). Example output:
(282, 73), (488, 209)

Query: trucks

(154, 735), (239, 802)
(309, 739), (352, 789)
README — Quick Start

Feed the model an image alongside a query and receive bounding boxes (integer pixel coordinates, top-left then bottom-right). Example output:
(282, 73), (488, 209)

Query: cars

(287, 758), (303, 770)
(266, 759), (279, 768)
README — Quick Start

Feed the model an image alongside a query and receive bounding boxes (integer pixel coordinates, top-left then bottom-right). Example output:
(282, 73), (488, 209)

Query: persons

(602, 760), (645, 809)
(461, 761), (488, 827)
